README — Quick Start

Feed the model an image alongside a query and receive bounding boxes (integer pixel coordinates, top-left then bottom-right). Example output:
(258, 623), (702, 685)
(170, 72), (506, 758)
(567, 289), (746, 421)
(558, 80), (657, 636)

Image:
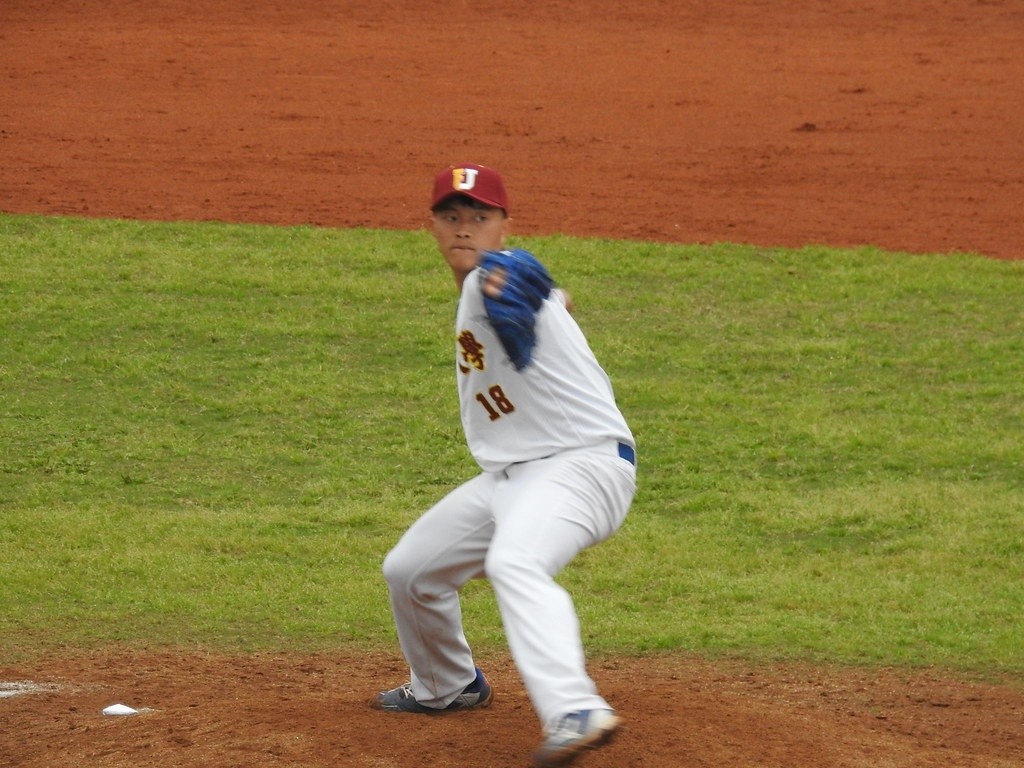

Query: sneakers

(369, 666), (494, 712)
(534, 709), (619, 760)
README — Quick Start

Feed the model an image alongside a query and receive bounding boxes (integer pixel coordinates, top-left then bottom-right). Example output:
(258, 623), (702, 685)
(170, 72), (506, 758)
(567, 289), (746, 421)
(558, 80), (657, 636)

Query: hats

(429, 164), (509, 220)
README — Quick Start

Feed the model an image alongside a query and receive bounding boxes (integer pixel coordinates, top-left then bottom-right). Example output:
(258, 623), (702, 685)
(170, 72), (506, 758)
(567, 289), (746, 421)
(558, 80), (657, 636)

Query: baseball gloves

(473, 246), (558, 374)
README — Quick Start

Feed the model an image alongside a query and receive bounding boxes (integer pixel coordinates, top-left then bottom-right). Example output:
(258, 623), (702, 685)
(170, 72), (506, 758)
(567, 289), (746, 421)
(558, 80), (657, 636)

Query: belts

(619, 442), (637, 468)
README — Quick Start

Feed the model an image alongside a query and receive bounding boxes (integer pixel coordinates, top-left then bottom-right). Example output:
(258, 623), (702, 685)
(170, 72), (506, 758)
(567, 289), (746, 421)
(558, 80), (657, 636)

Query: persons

(375, 162), (637, 763)
